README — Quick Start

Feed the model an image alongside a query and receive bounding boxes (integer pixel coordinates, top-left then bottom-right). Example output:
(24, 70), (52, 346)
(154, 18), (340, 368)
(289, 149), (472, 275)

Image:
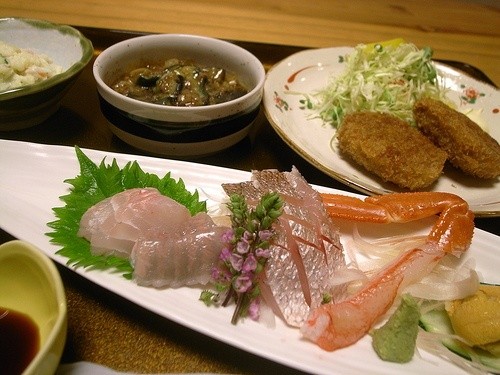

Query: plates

(0, 140), (500, 375)
(264, 46), (500, 217)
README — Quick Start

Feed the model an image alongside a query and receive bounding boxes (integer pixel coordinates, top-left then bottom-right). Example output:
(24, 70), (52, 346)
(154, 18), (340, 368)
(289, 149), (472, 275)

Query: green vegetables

(44, 145), (209, 280)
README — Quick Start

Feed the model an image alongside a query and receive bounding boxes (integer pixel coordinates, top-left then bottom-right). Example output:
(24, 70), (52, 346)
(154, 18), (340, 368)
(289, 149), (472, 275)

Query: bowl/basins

(0, 240), (68, 375)
(0, 16), (94, 132)
(94, 33), (266, 160)
